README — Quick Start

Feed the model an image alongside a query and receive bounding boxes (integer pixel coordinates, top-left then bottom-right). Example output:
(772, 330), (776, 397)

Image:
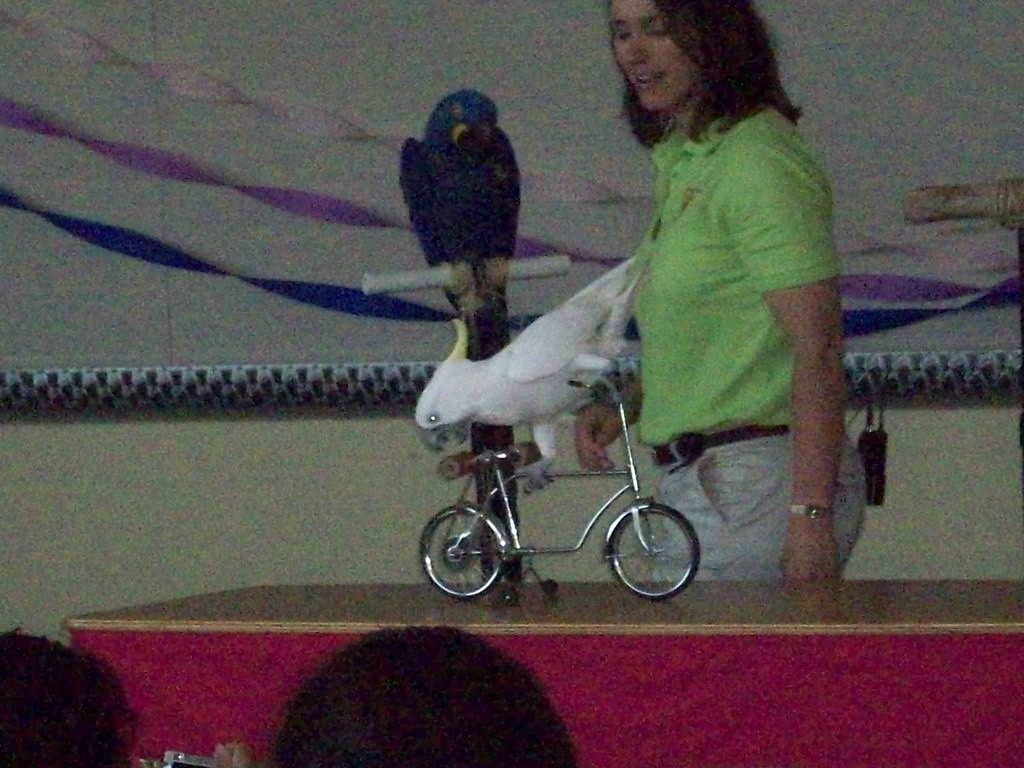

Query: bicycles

(417, 377), (701, 606)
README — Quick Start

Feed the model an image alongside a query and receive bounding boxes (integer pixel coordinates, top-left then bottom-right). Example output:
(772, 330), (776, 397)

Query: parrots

(414, 243), (649, 466)
(396, 87), (524, 594)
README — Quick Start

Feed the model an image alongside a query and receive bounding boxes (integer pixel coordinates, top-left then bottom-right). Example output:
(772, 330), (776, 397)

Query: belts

(654, 423), (790, 466)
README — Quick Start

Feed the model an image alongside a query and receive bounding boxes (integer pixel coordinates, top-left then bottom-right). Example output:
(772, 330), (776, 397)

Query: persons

(268, 623), (574, 768)
(575, 2), (870, 594)
(0, 628), (133, 768)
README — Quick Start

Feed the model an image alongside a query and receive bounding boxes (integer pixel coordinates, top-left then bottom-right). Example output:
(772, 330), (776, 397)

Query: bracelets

(788, 503), (832, 521)
